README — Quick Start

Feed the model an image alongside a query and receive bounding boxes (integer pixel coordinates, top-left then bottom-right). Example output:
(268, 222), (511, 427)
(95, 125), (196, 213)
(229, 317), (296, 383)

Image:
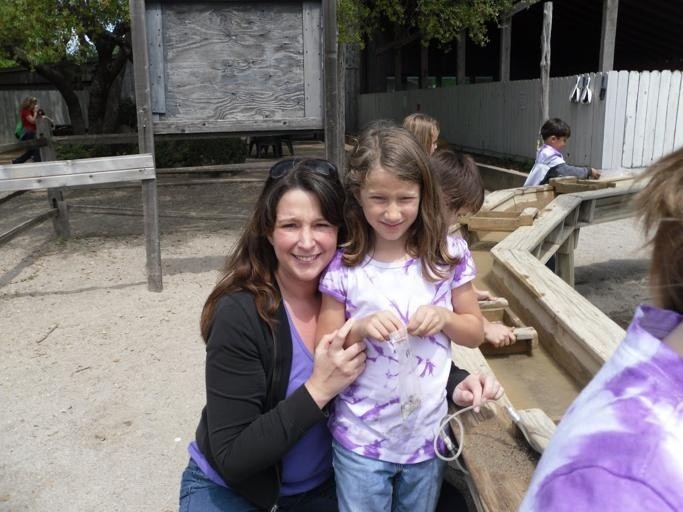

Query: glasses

(270, 158), (336, 180)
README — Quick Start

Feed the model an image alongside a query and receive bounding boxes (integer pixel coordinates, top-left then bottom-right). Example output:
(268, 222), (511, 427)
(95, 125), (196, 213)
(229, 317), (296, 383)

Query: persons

(9, 95), (41, 163)
(177, 157), (506, 512)
(312, 115), (485, 512)
(420, 141), (519, 352)
(509, 146), (683, 511)
(399, 108), (443, 157)
(521, 118), (601, 186)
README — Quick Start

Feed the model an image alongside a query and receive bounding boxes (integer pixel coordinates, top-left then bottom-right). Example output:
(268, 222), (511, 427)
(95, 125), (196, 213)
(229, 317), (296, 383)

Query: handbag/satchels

(16, 122), (24, 140)
